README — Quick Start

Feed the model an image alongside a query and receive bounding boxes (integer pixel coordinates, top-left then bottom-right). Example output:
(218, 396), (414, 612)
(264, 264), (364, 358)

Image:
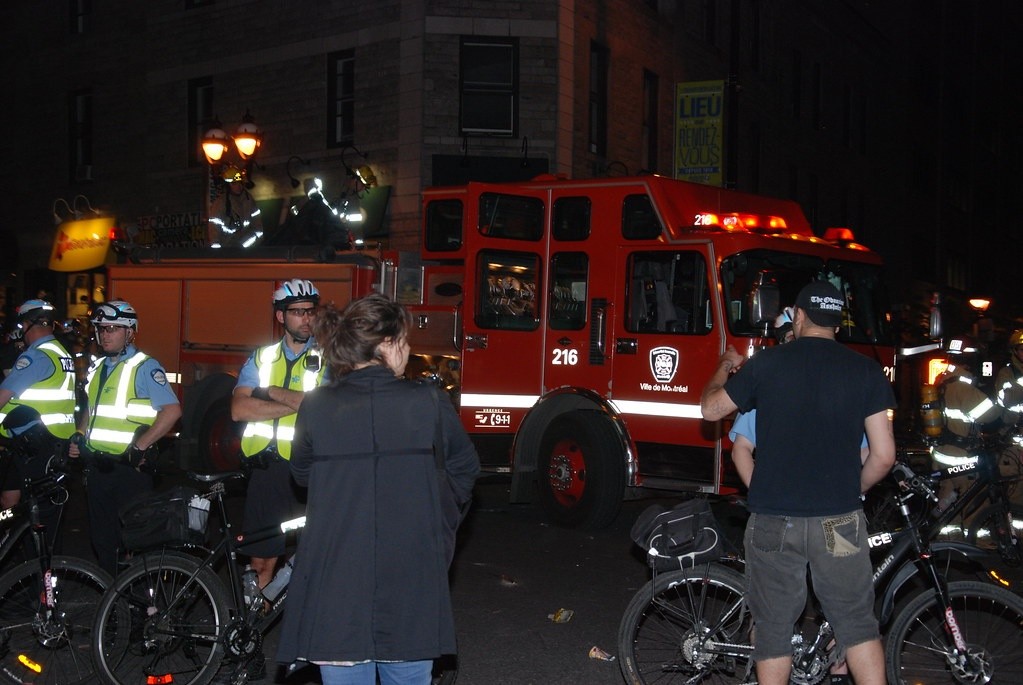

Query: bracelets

(76, 429), (85, 436)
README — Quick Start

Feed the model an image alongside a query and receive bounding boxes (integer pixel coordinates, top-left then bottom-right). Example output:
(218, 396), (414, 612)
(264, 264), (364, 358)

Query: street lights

(200, 109), (265, 235)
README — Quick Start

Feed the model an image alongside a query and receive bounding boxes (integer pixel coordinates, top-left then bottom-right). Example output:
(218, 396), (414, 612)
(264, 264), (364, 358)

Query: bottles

(930, 486), (961, 517)
(262, 554), (296, 601)
(241, 564), (264, 611)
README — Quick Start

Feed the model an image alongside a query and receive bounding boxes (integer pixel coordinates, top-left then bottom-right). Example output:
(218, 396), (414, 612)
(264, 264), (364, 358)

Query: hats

(795, 281), (844, 328)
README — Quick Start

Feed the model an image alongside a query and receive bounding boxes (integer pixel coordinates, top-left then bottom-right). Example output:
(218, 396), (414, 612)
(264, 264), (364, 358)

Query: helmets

(946, 336), (978, 354)
(274, 278), (319, 308)
(89, 300), (139, 332)
(18, 299), (57, 322)
(1009, 329), (1023, 348)
(774, 307), (794, 336)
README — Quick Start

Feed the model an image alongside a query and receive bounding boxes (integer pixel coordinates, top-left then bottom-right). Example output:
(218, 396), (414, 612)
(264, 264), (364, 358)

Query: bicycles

(866, 422), (1023, 626)
(0, 427), (131, 685)
(89, 470), (307, 685)
(618, 461), (1023, 685)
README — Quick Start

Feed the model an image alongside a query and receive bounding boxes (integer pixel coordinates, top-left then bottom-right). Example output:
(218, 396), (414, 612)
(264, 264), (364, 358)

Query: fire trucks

(48, 174), (896, 529)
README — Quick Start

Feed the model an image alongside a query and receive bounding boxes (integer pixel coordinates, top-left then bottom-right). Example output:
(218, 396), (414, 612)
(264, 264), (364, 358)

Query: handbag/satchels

(632, 491), (747, 573)
(123, 496), (190, 548)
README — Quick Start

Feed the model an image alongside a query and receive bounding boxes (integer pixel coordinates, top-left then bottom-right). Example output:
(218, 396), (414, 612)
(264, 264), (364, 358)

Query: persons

(274, 290), (483, 685)
(66, 299), (185, 649)
(212, 276), (337, 685)
(0, 288), (78, 610)
(205, 163), (268, 252)
(287, 163), (377, 247)
(697, 279), (899, 685)
(916, 327), (1023, 608)
(815, 262), (862, 341)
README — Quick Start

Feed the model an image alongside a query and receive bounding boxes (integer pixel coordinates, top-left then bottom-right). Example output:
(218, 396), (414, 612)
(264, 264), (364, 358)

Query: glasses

(97, 325), (126, 334)
(287, 307), (318, 317)
(93, 303), (136, 321)
